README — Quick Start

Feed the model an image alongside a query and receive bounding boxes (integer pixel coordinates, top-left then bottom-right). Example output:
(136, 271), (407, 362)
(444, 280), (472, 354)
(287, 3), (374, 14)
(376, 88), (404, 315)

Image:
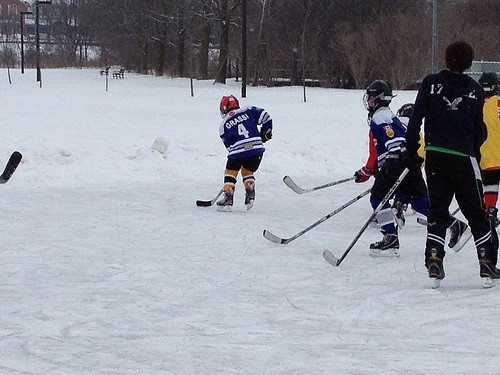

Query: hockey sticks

(417, 205), (460, 226)
(322, 167), (409, 267)
(0, 151), (22, 184)
(262, 188), (372, 244)
(196, 128), (271, 207)
(284, 175), (357, 195)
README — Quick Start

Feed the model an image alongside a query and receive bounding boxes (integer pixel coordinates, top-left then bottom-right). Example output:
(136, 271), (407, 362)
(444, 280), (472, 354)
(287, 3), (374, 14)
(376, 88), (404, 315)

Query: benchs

(112, 68), (126, 78)
(100, 66), (111, 75)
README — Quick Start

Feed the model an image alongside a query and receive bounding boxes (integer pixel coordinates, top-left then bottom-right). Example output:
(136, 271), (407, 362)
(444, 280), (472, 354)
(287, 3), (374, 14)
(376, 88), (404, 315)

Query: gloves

(398, 141), (424, 172)
(382, 153), (400, 176)
(354, 167), (374, 183)
(260, 124), (272, 142)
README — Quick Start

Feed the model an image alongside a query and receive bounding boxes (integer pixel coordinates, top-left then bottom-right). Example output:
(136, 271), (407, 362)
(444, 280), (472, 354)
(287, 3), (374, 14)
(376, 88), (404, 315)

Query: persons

(386, 39), (500, 283)
(471, 70), (500, 231)
(368, 98), (425, 229)
(215, 93), (274, 209)
(358, 78), (470, 254)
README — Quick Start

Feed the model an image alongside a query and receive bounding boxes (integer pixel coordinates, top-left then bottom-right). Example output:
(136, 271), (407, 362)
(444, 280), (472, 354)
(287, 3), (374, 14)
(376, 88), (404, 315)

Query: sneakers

(369, 226), (401, 258)
(448, 218), (473, 253)
(216, 190), (234, 213)
(371, 200), (406, 225)
(425, 246), (445, 289)
(477, 244), (500, 288)
(488, 206), (500, 226)
(244, 182), (256, 211)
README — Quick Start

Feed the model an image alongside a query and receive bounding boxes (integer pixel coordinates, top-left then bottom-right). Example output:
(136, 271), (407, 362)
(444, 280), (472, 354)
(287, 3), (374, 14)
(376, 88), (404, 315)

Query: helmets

(219, 94), (241, 118)
(399, 103), (415, 117)
(478, 71), (500, 95)
(366, 79), (393, 106)
